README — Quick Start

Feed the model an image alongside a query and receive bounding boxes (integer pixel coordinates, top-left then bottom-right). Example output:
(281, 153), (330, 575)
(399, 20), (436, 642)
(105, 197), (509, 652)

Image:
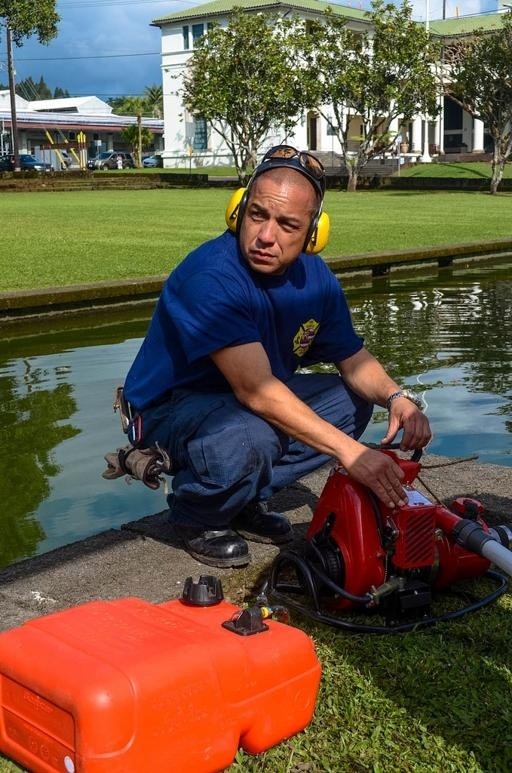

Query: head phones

(225, 159), (330, 256)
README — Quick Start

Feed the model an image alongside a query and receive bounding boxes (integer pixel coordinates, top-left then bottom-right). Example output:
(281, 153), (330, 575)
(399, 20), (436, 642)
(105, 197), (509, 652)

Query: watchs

(387, 387), (423, 410)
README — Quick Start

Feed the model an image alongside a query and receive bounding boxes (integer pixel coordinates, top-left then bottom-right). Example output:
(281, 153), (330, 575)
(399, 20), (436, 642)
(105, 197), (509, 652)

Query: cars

(87, 150), (134, 170)
(0, 154), (52, 172)
(61, 151), (72, 170)
(142, 154), (162, 168)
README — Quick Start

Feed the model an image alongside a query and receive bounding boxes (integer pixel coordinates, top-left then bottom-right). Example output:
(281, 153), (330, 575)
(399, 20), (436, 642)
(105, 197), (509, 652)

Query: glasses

(261, 145), (325, 180)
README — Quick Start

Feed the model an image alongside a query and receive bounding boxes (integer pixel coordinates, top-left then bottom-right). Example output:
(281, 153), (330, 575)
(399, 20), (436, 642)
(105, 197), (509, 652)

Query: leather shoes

(163, 497), (293, 567)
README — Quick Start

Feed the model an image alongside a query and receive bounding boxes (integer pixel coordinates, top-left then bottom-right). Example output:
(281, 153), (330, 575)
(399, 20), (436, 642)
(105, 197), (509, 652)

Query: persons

(119, 144), (431, 567)
